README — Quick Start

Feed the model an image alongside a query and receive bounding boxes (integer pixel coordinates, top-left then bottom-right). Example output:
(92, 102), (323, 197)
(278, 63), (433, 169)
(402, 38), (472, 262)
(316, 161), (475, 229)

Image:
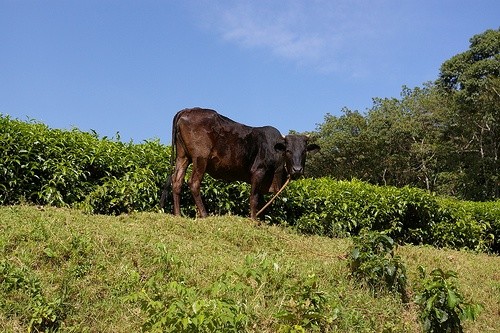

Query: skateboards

(159, 107), (321, 220)
